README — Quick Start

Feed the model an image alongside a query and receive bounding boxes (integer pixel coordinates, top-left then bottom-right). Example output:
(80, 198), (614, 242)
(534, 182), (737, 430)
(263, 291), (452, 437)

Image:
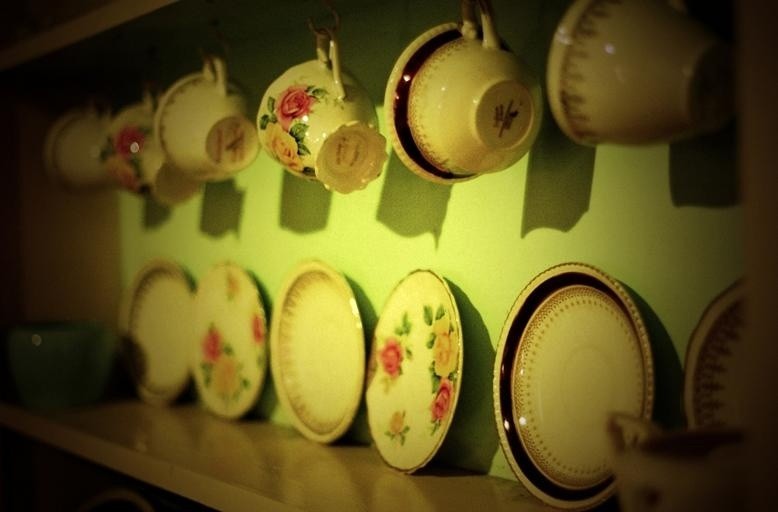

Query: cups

(95, 100), (194, 202)
(547, 1), (731, 150)
(384, 2), (544, 186)
(7, 320), (113, 411)
(151, 55), (260, 185)
(47, 108), (113, 194)
(258, 31), (390, 196)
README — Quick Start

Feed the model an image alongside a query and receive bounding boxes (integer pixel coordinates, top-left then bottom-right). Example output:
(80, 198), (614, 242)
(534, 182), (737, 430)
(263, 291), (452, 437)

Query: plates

(683, 272), (778, 460)
(124, 256), (202, 410)
(367, 267), (464, 475)
(271, 260), (366, 447)
(188, 260), (269, 423)
(491, 263), (656, 512)
